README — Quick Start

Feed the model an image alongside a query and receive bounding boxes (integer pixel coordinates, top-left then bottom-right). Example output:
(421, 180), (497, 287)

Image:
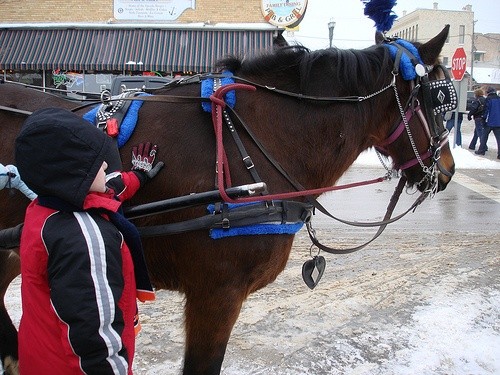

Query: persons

(443, 112), (463, 149)
(14, 107), (164, 375)
(475, 87), (500, 159)
(468, 89), (488, 152)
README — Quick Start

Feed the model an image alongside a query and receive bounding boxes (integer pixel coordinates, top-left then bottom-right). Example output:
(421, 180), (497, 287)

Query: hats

(486, 87), (494, 93)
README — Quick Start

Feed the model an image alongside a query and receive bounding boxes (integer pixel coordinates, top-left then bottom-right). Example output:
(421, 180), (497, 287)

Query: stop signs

(451, 47), (467, 81)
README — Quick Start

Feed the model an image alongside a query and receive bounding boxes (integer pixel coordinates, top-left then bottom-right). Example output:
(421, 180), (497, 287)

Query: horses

(0, 25), (455, 375)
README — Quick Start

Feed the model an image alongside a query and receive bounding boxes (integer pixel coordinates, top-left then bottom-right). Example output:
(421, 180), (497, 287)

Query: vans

(110, 76), (173, 97)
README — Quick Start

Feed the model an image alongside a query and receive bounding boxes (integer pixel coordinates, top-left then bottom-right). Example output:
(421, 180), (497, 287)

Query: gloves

(130, 142), (164, 188)
(481, 119), (485, 123)
(468, 115), (471, 120)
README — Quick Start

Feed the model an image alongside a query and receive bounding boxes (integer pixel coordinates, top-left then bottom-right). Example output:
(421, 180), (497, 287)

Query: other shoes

(469, 146), (475, 150)
(498, 156), (500, 159)
(475, 151), (485, 155)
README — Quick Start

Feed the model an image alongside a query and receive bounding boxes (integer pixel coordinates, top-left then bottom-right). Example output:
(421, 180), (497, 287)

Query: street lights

(328, 16), (336, 46)
